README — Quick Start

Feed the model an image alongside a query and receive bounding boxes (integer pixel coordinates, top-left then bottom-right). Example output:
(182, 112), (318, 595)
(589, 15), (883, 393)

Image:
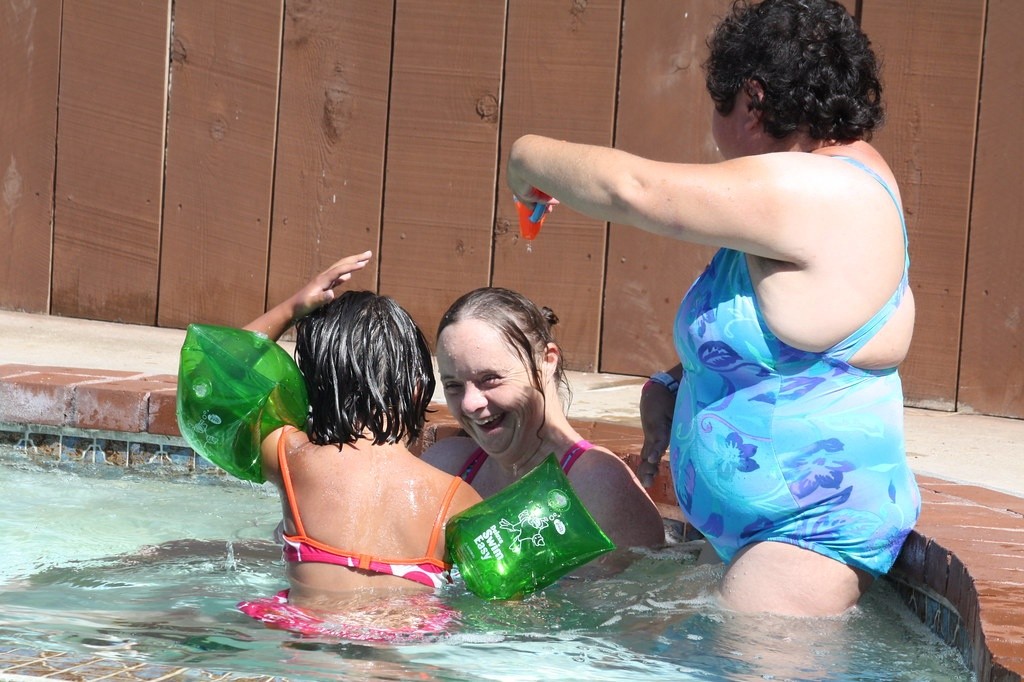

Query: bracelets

(646, 371), (679, 397)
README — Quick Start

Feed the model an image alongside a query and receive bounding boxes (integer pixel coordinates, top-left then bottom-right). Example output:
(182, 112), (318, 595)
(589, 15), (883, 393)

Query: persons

(507, 0), (922, 623)
(416, 286), (666, 558)
(179, 250), (483, 602)
